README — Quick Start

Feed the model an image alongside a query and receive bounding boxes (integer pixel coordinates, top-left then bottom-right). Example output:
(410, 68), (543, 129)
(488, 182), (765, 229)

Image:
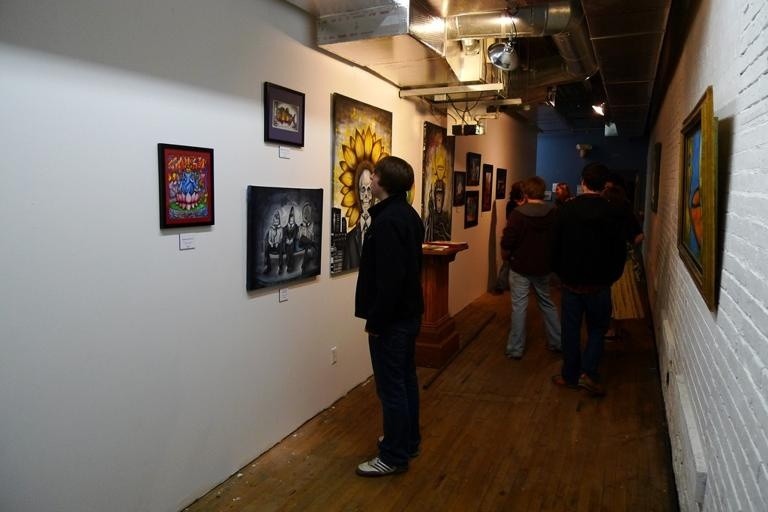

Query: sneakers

(377, 434), (421, 457)
(356, 453), (408, 477)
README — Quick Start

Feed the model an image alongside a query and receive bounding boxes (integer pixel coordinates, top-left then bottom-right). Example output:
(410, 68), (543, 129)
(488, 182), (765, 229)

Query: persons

(263, 201), (319, 273)
(500, 160), (645, 396)
(355, 157), (425, 477)
(342, 169), (372, 271)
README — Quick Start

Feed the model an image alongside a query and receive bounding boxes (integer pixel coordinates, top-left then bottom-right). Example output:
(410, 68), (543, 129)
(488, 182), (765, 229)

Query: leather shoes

(578, 372), (606, 394)
(552, 375), (567, 386)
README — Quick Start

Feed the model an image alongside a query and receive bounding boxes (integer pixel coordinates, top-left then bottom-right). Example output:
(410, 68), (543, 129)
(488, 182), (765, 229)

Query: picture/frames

(264, 82), (305, 147)
(677, 85), (718, 313)
(158, 144), (214, 229)
(651, 143), (661, 214)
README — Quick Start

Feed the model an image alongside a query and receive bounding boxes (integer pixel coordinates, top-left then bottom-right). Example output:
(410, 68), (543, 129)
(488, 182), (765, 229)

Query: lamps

(592, 102), (606, 118)
(488, 33), (519, 71)
